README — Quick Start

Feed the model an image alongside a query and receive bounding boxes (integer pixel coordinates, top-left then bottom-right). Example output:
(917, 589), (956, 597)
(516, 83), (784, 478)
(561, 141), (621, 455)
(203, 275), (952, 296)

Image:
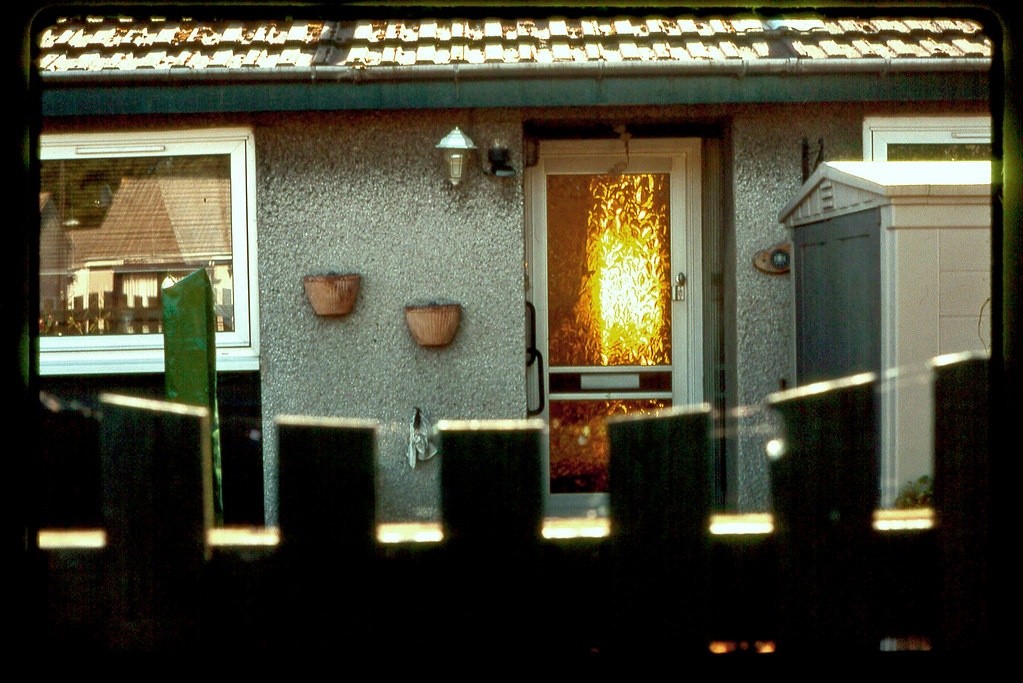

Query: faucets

(413, 406), (422, 428)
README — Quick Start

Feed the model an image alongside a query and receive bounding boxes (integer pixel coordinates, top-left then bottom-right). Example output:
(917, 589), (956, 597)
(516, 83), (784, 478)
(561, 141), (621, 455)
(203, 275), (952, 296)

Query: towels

(409, 412), (438, 468)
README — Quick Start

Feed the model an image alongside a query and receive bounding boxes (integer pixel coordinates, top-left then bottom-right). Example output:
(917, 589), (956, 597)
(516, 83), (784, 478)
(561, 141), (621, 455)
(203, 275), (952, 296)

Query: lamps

(435, 126), (516, 189)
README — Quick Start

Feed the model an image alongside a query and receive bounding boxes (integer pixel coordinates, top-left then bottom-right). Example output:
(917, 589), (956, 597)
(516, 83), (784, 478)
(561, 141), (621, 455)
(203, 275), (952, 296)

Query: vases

(403, 303), (462, 346)
(302, 272), (361, 316)
